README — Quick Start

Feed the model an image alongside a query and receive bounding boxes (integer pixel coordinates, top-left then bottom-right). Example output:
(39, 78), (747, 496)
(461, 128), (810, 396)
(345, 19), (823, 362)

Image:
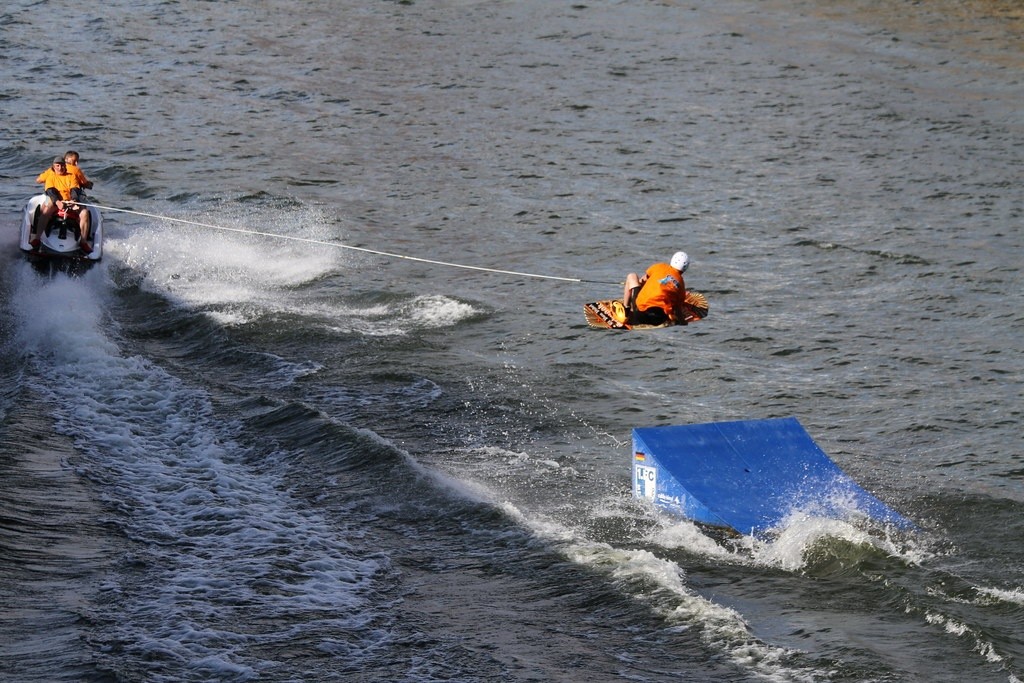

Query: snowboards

(584, 293), (709, 329)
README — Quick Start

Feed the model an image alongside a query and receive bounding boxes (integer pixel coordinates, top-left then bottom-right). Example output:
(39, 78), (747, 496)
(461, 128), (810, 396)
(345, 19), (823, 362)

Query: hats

(53, 156), (66, 164)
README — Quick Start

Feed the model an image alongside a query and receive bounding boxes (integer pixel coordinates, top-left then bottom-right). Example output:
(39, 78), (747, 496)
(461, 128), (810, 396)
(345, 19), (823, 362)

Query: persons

(30, 150), (93, 254)
(623, 251), (690, 321)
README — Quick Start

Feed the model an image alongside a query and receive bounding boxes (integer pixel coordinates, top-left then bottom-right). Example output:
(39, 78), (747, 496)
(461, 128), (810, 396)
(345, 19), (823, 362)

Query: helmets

(670, 252), (690, 272)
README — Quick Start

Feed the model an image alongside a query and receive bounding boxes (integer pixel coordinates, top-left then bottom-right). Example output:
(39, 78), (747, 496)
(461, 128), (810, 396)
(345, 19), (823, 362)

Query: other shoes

(30, 238), (41, 245)
(622, 303), (631, 315)
(79, 240), (93, 253)
